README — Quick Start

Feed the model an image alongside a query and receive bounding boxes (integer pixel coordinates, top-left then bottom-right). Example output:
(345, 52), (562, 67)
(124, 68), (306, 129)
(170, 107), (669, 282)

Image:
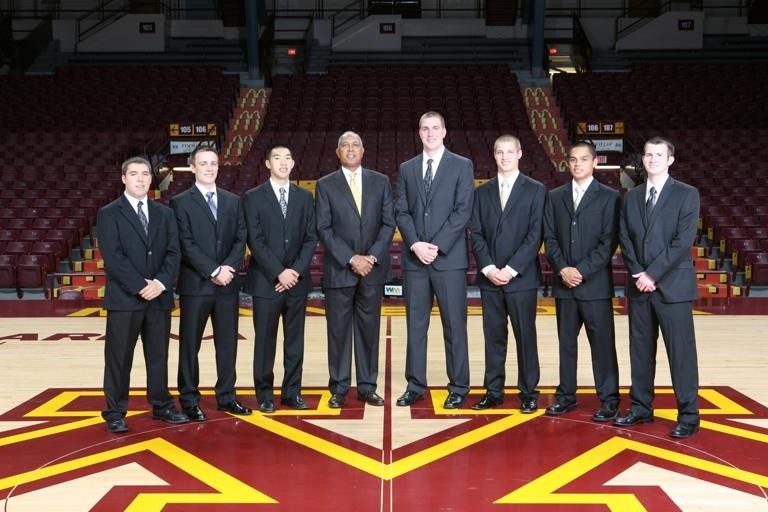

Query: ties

(280, 188), (288, 218)
(574, 188), (585, 209)
(500, 181), (508, 213)
(646, 186), (656, 225)
(348, 172), (360, 215)
(138, 201), (148, 236)
(206, 192), (218, 222)
(424, 160), (432, 198)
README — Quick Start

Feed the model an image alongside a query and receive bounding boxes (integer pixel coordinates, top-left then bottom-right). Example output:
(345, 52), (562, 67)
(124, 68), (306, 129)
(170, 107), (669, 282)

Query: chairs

(0, 62), (768, 300)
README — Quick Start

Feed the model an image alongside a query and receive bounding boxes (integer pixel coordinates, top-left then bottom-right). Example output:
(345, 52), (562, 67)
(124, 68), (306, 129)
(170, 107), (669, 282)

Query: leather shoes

(107, 419), (128, 432)
(328, 391), (344, 407)
(152, 396), (306, 422)
(357, 391), (387, 405)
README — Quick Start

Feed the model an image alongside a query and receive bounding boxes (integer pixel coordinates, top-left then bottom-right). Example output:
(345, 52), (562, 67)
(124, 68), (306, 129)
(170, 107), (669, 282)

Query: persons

(394, 111), (474, 410)
(542, 142), (621, 423)
(613, 135), (700, 439)
(242, 143), (319, 414)
(314, 130), (397, 409)
(168, 146), (252, 422)
(97, 156), (190, 433)
(470, 135), (547, 414)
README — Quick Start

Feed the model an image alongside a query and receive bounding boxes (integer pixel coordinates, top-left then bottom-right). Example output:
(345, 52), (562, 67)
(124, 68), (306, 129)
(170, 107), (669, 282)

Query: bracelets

(214, 267), (221, 277)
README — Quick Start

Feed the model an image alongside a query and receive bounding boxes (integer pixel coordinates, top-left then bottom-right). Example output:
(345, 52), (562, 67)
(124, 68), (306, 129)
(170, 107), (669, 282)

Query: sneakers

(545, 397), (577, 415)
(593, 403), (621, 421)
(670, 422), (699, 438)
(614, 413), (654, 426)
(520, 397), (538, 414)
(471, 394), (502, 409)
(397, 391), (422, 406)
(444, 393), (466, 409)
